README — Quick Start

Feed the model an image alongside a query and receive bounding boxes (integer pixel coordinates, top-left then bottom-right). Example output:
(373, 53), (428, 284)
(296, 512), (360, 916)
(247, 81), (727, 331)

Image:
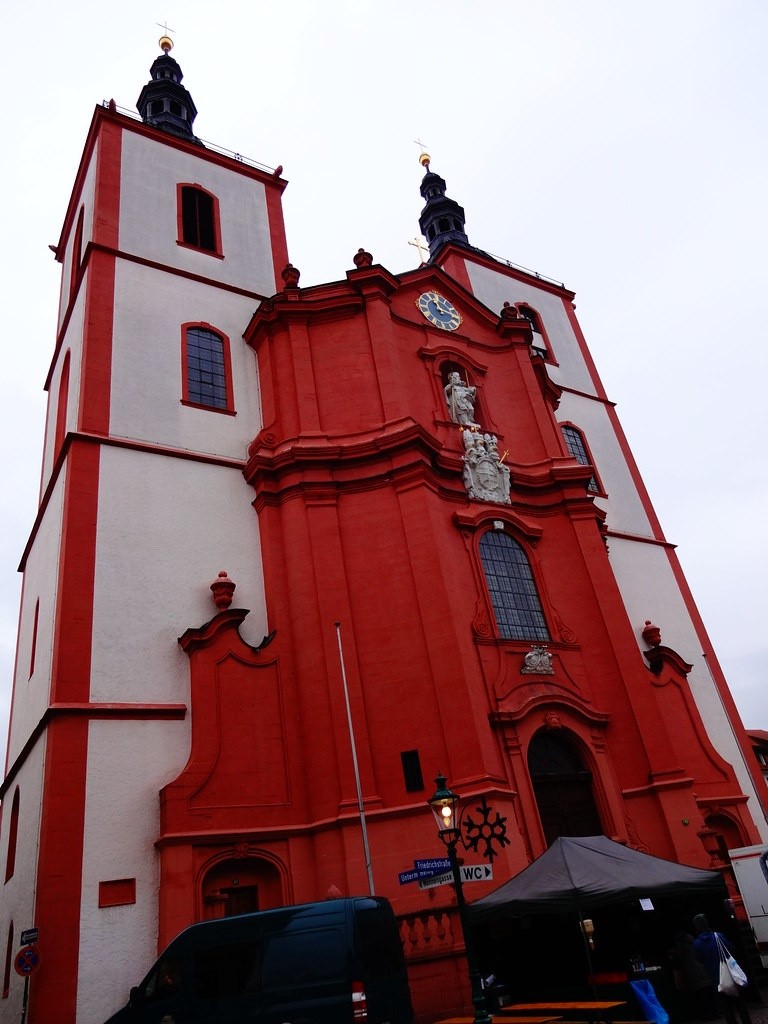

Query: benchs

(544, 1020), (657, 1024)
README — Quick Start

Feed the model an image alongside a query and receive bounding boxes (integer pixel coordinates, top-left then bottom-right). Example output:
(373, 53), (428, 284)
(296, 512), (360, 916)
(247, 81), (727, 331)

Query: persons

(443, 371), (482, 428)
(691, 913), (756, 1024)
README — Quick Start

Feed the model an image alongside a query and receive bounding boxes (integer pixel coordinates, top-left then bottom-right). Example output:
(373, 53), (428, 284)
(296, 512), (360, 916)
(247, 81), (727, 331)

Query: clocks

(415, 290), (464, 332)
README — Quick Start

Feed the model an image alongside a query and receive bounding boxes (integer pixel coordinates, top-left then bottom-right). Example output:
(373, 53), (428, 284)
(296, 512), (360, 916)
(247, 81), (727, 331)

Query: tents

(463, 833), (744, 1024)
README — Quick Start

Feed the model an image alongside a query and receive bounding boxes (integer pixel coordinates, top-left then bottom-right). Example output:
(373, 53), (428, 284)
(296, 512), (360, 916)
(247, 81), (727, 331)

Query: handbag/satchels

(709, 932), (739, 997)
(715, 932), (747, 986)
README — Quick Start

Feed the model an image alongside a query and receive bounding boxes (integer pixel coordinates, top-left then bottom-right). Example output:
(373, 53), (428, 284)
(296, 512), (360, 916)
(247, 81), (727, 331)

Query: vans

(104, 895), (414, 1024)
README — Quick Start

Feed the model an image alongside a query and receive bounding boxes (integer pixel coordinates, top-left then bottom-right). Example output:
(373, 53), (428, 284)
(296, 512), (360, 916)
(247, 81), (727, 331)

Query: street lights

(426, 770), (494, 1024)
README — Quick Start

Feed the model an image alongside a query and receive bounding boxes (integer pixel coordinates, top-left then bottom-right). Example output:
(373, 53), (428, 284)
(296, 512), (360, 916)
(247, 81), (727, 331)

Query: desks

(432, 1000), (625, 1024)
